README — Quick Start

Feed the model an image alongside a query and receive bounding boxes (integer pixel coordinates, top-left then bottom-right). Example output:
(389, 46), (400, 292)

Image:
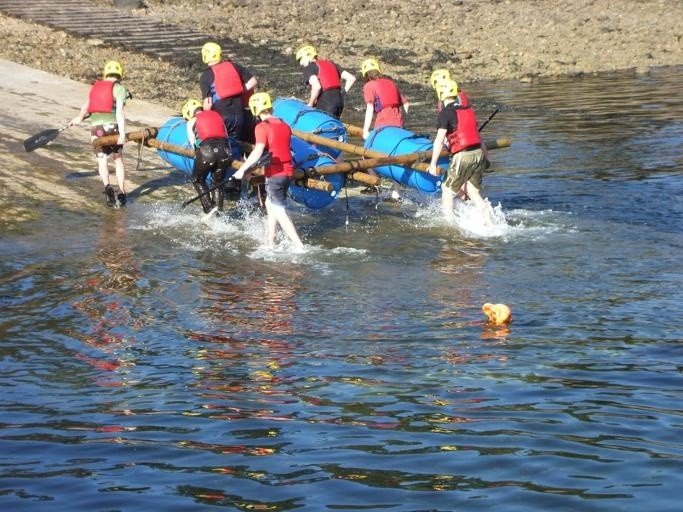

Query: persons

(295, 46), (355, 120)
(359, 60), (409, 194)
(426, 79), (486, 219)
(67, 60), (127, 206)
(233, 92), (303, 250)
(429, 69), (467, 110)
(199, 41), (257, 199)
(181, 100), (230, 215)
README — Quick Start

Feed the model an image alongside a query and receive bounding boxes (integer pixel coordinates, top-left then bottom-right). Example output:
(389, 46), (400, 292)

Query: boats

(146, 94), (458, 213)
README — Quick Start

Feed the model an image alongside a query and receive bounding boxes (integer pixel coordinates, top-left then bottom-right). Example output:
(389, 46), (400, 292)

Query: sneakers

(103, 184), (116, 208)
(381, 196), (404, 204)
(358, 185), (383, 196)
(199, 206), (220, 223)
(117, 192), (127, 206)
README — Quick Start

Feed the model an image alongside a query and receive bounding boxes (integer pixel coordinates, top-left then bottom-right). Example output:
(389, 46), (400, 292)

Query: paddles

(24, 91), (132, 152)
(182, 152), (272, 207)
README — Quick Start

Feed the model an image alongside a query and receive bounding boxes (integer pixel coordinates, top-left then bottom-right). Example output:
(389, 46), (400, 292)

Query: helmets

(247, 92), (273, 117)
(360, 58), (382, 78)
(102, 60), (124, 80)
(180, 98), (205, 122)
(295, 45), (318, 65)
(430, 68), (458, 102)
(201, 41), (223, 65)
